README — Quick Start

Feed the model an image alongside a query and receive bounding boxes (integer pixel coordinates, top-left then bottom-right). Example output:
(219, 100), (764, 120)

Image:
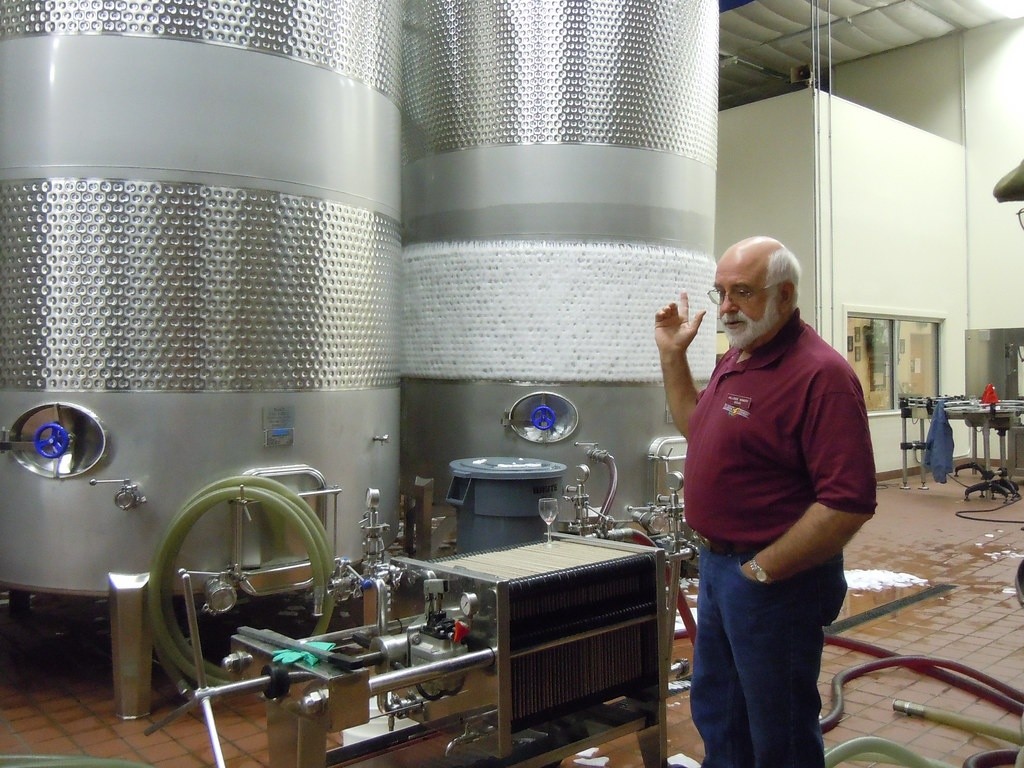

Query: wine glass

(538, 498), (559, 549)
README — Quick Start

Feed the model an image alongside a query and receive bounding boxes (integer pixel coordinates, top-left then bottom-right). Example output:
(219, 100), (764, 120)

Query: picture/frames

(855, 346), (861, 361)
(900, 339), (905, 353)
(847, 336), (853, 351)
(855, 327), (860, 342)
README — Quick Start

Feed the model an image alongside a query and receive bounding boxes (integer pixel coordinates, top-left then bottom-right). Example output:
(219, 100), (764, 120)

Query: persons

(655, 236), (878, 768)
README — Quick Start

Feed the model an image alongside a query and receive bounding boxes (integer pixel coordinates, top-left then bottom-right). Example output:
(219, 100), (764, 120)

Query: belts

(698, 536), (753, 554)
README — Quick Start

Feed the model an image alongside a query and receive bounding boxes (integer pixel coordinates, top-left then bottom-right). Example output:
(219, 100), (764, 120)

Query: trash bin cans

(445, 456), (568, 555)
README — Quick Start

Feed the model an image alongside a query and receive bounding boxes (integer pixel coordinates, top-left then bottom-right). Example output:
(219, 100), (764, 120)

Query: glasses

(706, 280), (784, 306)
(1016, 206), (1023, 229)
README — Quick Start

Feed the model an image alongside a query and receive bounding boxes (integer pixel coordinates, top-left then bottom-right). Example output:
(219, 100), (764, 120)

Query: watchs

(750, 557), (774, 584)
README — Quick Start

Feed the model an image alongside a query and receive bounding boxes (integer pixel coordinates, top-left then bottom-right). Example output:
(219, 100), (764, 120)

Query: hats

(993, 159), (1024, 203)
